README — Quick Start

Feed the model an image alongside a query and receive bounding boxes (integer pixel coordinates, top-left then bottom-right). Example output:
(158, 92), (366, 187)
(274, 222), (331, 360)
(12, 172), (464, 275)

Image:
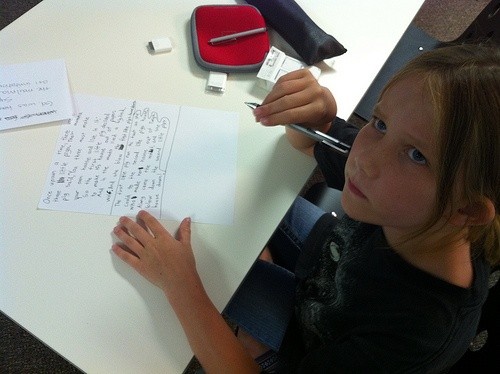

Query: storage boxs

(257, 45), (321, 92)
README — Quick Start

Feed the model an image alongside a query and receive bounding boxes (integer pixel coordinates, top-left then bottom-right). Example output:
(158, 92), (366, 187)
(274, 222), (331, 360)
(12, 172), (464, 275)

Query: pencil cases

(246, 0), (351, 62)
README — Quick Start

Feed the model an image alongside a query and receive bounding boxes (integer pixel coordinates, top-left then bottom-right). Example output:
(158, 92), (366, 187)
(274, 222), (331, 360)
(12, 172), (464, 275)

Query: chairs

(354, 0), (500, 123)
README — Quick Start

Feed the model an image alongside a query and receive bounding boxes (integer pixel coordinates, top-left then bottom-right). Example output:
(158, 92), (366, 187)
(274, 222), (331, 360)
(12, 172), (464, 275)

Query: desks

(0, 0), (426, 374)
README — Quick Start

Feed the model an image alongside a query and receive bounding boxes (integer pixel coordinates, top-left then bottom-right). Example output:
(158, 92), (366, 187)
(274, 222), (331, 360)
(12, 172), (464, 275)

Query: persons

(111, 46), (499, 374)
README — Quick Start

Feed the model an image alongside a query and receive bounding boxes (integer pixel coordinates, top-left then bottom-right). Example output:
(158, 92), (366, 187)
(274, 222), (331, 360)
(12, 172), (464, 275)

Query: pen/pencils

(248, 102), (353, 154)
(208, 25), (268, 47)
